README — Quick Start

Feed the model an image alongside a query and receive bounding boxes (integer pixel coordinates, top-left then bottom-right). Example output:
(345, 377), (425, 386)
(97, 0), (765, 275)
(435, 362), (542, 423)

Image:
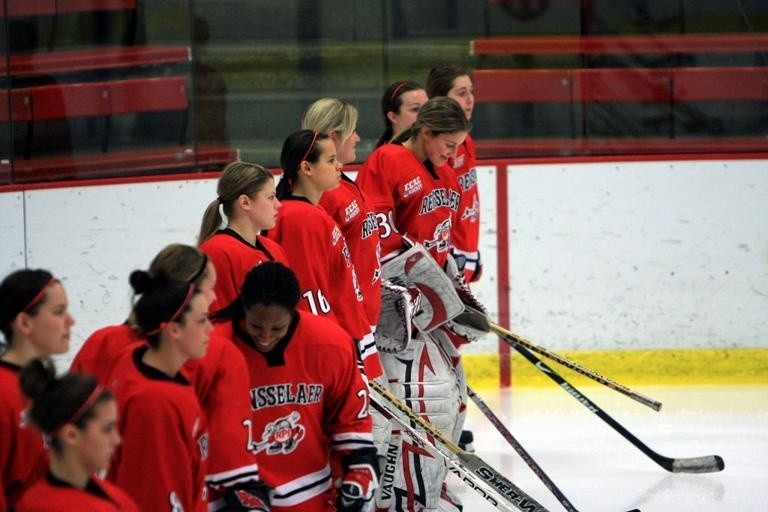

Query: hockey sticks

(502, 339), (726, 474)
(468, 385), (640, 512)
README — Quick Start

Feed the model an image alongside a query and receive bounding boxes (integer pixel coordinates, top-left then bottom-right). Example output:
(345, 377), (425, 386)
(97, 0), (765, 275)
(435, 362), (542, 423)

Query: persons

(1, 267), (74, 509)
(196, 65), (480, 512)
(66, 242), (268, 512)
(15, 359), (139, 512)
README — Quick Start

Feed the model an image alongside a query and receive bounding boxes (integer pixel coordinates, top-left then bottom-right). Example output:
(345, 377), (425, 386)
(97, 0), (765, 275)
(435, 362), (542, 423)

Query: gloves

(336, 447), (380, 512)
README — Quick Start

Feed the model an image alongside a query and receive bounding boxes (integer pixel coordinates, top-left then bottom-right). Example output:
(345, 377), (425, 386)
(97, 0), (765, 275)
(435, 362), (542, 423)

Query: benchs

(465, 34), (768, 159)
(0, 0), (237, 186)
